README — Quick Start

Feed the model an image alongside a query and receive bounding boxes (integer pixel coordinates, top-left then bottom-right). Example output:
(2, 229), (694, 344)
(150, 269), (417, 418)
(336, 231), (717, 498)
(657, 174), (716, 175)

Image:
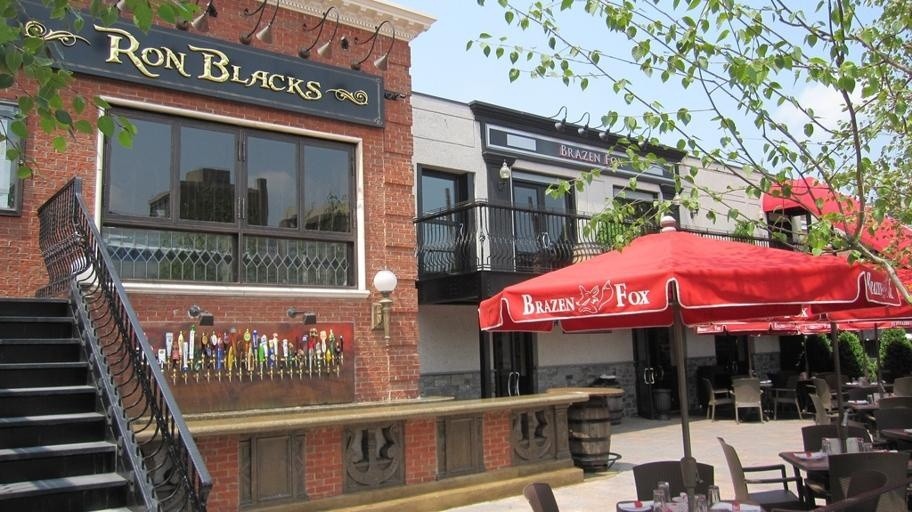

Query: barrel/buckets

(590, 381), (624, 426)
(567, 400), (613, 473)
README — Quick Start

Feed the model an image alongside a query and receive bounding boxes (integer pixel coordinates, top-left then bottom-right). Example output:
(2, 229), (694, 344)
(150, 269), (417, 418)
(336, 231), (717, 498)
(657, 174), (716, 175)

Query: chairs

(700, 367), (911, 512)
(632, 462), (715, 501)
(523, 482), (559, 511)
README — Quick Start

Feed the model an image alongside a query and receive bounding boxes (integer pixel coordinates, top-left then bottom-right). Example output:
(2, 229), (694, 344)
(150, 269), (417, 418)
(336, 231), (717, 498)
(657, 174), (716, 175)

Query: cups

(679, 492), (707, 512)
(708, 484), (720, 505)
(821, 435), (871, 454)
(652, 480), (671, 511)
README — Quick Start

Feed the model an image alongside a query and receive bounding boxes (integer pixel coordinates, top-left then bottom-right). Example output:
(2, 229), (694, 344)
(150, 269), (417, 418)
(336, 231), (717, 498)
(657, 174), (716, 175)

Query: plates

(798, 451), (825, 461)
(619, 497), (753, 512)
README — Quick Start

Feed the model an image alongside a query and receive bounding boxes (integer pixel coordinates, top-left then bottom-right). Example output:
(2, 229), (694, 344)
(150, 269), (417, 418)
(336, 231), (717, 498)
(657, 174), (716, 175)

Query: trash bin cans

(652, 389), (672, 420)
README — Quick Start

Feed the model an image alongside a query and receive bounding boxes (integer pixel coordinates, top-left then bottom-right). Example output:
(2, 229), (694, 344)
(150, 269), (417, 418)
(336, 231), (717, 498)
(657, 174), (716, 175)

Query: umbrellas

(696, 268), (912, 453)
(477, 217), (901, 512)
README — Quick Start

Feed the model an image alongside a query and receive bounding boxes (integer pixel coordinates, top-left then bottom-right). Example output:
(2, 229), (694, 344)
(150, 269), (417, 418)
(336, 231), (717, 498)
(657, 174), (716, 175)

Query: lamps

(497, 158), (511, 191)
(239, 0), (281, 45)
(669, 192), (681, 212)
(288, 307), (317, 326)
(370, 269), (399, 348)
(547, 106), (653, 152)
(174, 0), (213, 34)
(351, 20), (396, 73)
(298, 6), (340, 60)
(190, 305), (215, 326)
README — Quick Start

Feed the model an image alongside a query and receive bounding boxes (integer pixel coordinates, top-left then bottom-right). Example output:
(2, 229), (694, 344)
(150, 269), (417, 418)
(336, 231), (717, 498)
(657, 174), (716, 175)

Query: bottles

(157, 321), (344, 372)
